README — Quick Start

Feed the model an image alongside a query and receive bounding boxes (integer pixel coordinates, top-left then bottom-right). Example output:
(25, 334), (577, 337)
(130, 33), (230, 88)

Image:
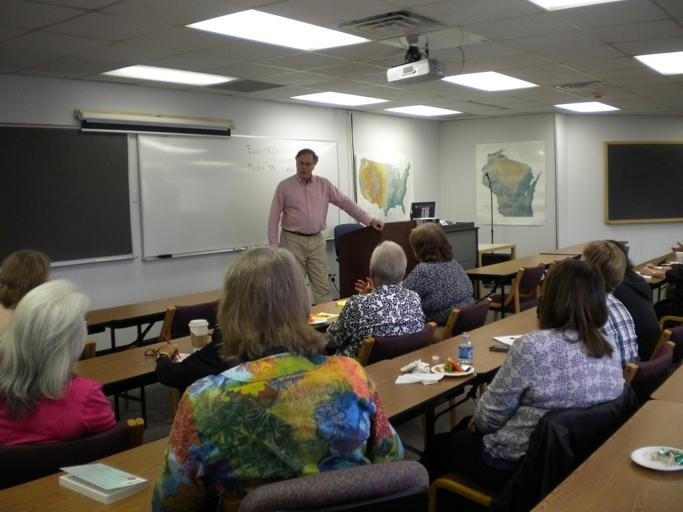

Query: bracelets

(156, 351), (171, 360)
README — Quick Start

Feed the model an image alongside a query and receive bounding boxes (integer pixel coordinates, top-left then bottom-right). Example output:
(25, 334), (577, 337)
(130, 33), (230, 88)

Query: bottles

(457, 332), (474, 366)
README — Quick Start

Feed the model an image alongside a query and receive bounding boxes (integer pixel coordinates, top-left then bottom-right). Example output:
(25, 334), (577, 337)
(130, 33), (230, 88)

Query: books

(57, 461), (149, 507)
(487, 333), (527, 354)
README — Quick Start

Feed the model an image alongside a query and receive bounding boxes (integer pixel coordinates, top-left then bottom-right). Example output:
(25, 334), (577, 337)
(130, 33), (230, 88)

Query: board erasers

(157, 254), (171, 258)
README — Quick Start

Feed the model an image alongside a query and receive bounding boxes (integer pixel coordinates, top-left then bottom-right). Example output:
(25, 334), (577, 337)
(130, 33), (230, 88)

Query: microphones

(485, 172), (491, 184)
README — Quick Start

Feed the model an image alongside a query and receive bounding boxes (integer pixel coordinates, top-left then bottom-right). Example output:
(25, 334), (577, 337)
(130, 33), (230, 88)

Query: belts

(281, 227), (322, 237)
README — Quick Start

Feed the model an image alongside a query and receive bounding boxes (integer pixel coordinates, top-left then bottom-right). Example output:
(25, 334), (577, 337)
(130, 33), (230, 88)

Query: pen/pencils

(160, 333), (183, 359)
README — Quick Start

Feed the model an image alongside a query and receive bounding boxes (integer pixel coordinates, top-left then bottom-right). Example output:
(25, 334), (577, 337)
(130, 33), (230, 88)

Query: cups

(188, 318), (209, 352)
(675, 251), (682, 263)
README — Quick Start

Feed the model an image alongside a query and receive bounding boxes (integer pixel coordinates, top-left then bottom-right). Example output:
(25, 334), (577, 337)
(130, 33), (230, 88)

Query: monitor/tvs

(411, 201), (435, 221)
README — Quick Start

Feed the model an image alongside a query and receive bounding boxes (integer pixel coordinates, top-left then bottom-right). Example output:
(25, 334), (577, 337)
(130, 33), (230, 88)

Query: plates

(430, 364), (474, 377)
(630, 446), (682, 472)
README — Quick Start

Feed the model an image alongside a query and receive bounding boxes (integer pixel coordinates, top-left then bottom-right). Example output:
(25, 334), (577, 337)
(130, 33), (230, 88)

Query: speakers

(481, 252), (517, 286)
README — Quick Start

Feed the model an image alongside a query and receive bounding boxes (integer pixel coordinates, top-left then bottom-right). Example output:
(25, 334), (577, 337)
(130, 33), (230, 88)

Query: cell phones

(489, 344), (510, 352)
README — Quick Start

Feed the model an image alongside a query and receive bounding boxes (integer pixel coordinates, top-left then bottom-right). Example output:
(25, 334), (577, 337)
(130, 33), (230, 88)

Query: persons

(150, 243), (407, 511)
(265, 148), (386, 309)
(670, 241), (683, 252)
(643, 259), (683, 328)
(155, 320), (246, 400)
(326, 238), (427, 364)
(608, 239), (666, 362)
(0, 248), (53, 338)
(0, 279), (119, 448)
(404, 221), (477, 328)
(575, 239), (640, 372)
(415, 256), (628, 511)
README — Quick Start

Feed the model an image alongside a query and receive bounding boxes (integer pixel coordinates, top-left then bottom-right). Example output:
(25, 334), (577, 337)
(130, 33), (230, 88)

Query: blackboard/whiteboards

(0, 122), (140, 268)
(135, 132), (341, 262)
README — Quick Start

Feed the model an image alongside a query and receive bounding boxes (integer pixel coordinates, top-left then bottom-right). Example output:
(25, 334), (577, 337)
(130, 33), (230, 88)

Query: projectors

(386, 58), (448, 83)
